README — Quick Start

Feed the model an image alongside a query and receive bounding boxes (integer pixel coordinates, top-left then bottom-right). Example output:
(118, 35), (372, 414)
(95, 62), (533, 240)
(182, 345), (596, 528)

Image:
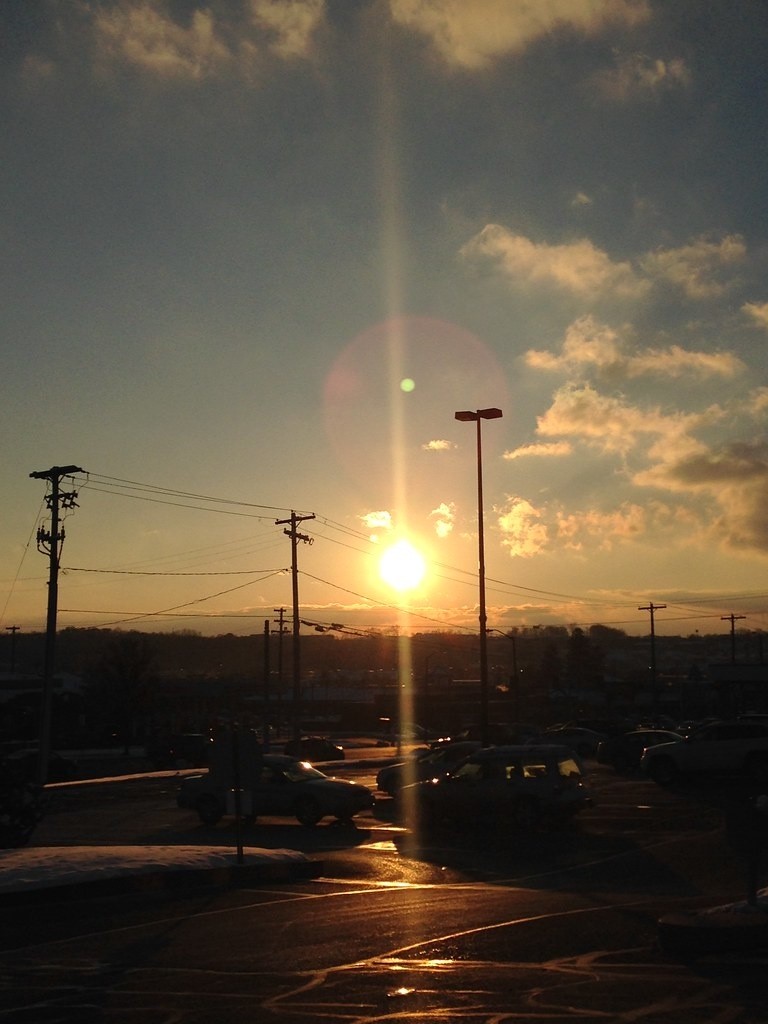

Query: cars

(287, 733), (346, 760)
(177, 752), (374, 826)
(375, 723), (684, 829)
(638, 713), (767, 784)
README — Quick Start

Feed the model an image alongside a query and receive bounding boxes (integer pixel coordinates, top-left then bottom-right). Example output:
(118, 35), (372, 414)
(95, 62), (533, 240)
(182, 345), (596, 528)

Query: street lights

(454, 406), (504, 720)
